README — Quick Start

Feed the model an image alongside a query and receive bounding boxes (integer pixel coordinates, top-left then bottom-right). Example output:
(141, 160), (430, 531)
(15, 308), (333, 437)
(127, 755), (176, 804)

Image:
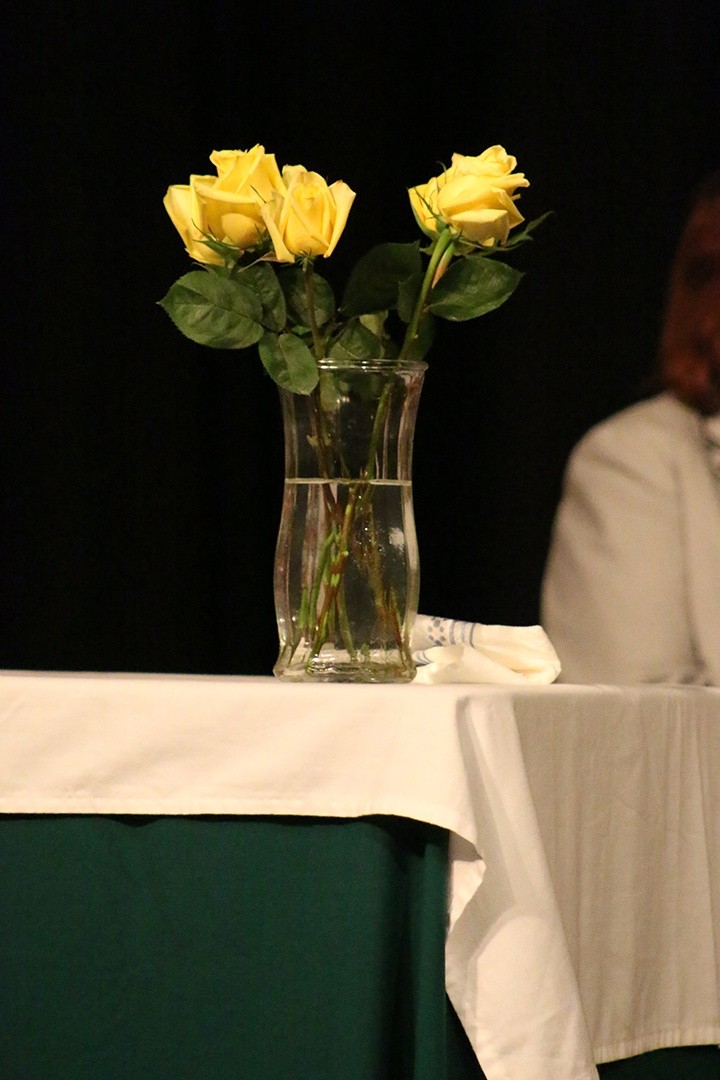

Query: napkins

(277, 613), (564, 685)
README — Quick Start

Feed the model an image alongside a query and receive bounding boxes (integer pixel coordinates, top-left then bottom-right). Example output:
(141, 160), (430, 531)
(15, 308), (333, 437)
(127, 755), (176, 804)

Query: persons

(542, 189), (720, 688)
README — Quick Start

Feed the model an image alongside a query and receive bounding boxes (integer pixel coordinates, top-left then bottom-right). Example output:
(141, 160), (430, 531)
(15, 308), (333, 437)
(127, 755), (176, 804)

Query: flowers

(147, 128), (564, 671)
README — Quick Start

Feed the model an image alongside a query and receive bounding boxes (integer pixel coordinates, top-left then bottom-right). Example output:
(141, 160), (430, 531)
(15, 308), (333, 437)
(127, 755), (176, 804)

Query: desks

(0, 657), (720, 1080)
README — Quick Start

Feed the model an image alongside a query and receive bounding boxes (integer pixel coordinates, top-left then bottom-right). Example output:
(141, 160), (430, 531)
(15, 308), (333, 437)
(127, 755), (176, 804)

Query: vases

(250, 356), (433, 685)
